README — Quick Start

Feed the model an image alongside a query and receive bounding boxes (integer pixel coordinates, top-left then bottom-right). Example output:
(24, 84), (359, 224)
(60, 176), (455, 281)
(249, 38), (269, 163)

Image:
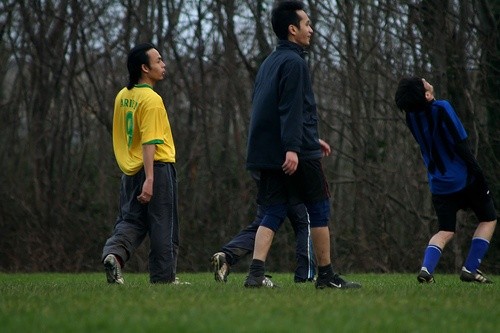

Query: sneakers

(104, 254), (124, 285)
(172, 277), (191, 286)
(311, 278), (317, 283)
(315, 272), (362, 290)
(211, 252), (230, 283)
(460, 266), (494, 284)
(417, 267), (435, 284)
(243, 274), (279, 289)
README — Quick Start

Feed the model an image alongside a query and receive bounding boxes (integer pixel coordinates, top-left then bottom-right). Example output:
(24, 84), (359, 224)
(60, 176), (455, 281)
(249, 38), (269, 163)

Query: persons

(395, 76), (497, 284)
(244, 0), (363, 290)
(101, 42), (192, 286)
(210, 169), (317, 284)
